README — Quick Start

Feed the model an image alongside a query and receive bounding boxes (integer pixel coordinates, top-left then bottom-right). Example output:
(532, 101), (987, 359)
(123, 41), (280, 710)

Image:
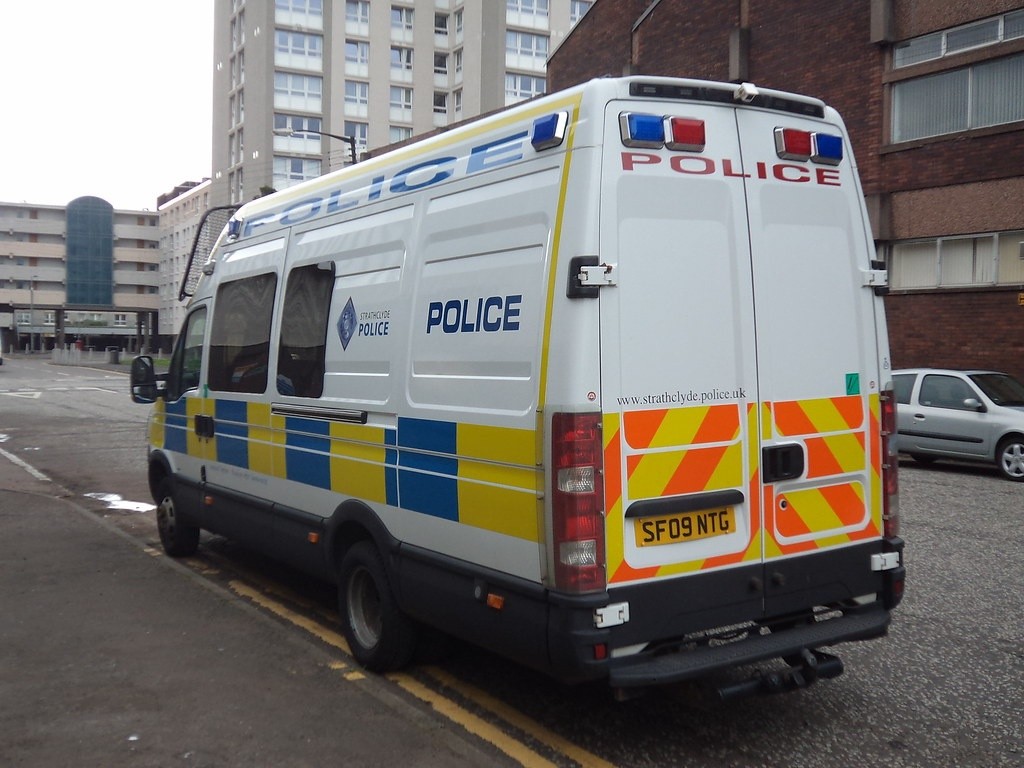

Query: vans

(130, 74), (908, 695)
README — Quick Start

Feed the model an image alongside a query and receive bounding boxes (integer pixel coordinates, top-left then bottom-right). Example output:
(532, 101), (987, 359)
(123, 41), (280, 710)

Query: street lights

(295, 128), (356, 166)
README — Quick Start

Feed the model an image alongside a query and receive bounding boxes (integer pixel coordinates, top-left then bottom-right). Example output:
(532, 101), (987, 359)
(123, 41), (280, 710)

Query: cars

(887, 368), (1024, 481)
